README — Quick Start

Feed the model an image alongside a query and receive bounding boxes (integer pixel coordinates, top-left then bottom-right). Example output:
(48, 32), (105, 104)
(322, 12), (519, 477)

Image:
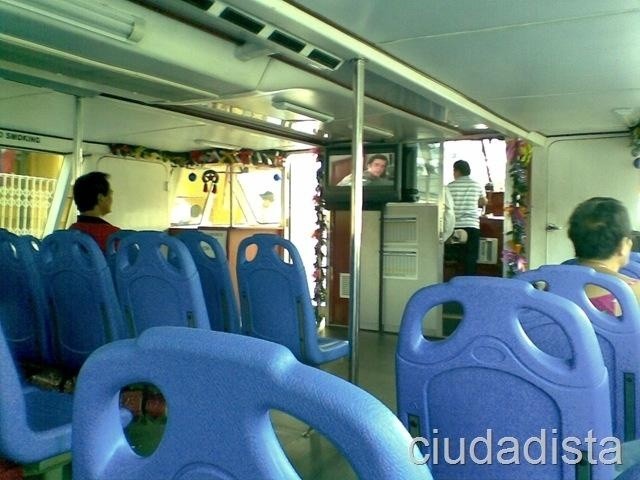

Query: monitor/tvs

(321, 141), (403, 211)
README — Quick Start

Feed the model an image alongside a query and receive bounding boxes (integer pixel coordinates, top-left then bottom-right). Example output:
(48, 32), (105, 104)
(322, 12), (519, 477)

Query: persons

(69, 170), (123, 255)
(416, 162), (457, 241)
(341, 154), (388, 186)
(535, 196), (640, 321)
(445, 161), (488, 276)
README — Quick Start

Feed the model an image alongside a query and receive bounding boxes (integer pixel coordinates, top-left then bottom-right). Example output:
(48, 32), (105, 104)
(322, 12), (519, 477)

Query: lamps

(347, 123), (394, 139)
(272, 102), (335, 124)
(1, 0), (147, 44)
